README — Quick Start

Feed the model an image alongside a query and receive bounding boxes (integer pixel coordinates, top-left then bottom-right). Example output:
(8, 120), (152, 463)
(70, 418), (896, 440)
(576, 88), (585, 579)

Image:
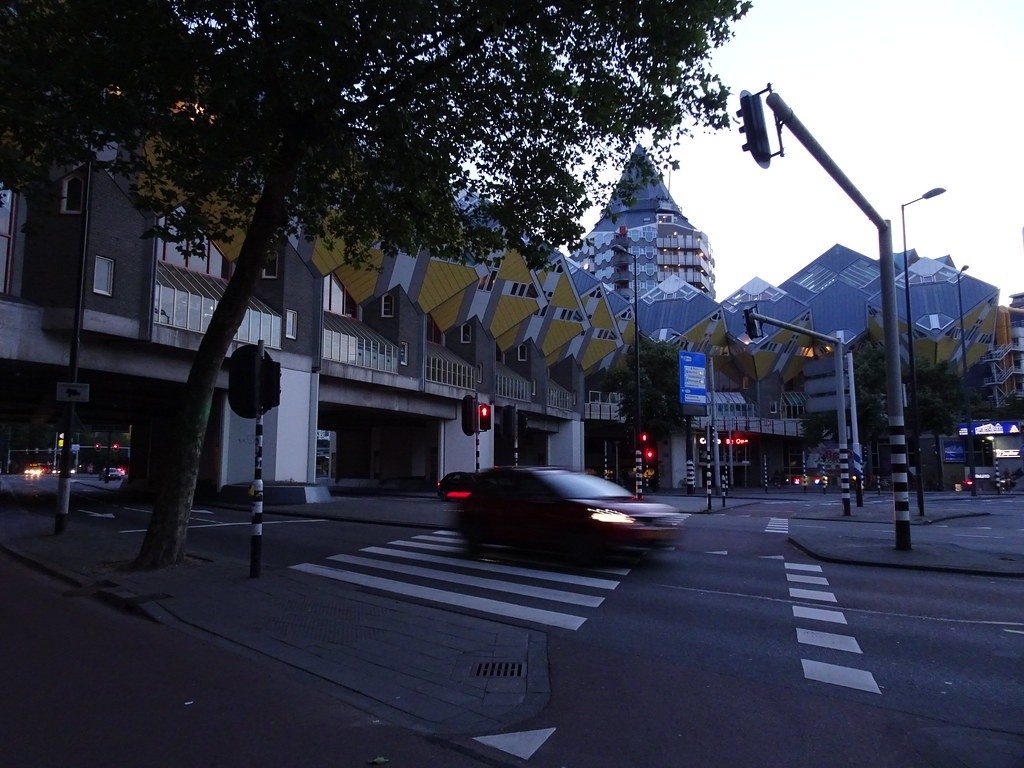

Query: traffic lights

(743, 305), (764, 339)
(640, 432), (648, 445)
(736, 88), (773, 171)
(477, 404), (492, 431)
(96, 442), (101, 452)
(645, 449), (655, 460)
(114, 443), (119, 452)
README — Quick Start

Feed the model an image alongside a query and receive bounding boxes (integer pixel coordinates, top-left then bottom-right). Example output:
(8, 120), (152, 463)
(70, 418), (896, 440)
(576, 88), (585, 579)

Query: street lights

(956, 264), (978, 497)
(900, 187), (948, 517)
(610, 244), (646, 501)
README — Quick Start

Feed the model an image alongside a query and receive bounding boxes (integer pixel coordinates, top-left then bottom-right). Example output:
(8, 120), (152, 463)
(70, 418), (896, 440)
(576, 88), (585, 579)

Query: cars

(117, 467), (125, 477)
(23, 464), (61, 476)
(437, 471), (475, 502)
(99, 468), (122, 481)
(455, 466), (683, 561)
